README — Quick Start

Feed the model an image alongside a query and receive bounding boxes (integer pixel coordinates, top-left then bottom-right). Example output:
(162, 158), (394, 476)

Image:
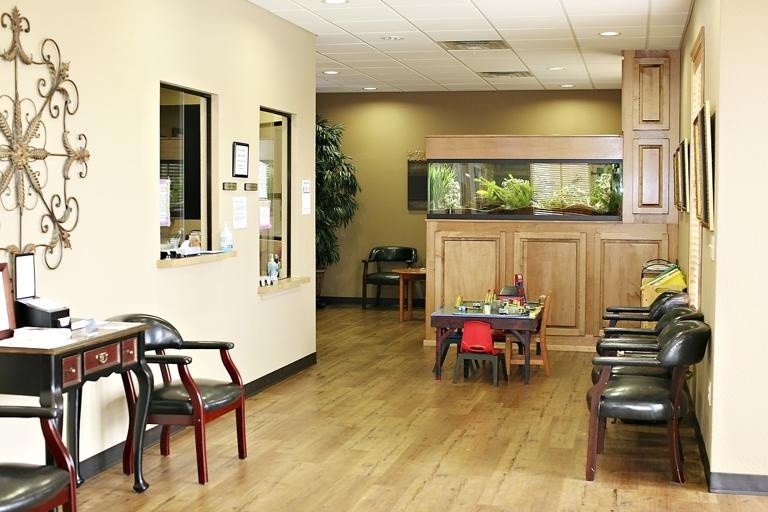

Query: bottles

(220, 223), (233, 251)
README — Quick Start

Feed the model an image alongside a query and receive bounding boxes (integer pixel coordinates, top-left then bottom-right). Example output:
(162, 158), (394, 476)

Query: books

(12, 327), (73, 342)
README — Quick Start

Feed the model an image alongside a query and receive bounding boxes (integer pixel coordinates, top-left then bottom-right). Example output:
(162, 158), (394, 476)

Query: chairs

(582, 289), (711, 487)
(102, 312), (247, 486)
(430, 289), (556, 388)
(0, 403), (78, 512)
(360, 242), (418, 316)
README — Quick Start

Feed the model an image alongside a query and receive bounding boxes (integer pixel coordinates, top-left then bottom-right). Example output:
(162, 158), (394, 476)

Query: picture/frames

(672, 97), (711, 231)
(232, 141), (249, 178)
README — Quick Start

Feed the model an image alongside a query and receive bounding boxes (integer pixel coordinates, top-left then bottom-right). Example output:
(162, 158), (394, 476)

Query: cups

(188, 235), (200, 251)
(168, 238), (178, 247)
(484, 304), (490, 314)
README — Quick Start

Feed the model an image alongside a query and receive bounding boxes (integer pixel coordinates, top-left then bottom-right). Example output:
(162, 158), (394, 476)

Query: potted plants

(316, 114), (366, 309)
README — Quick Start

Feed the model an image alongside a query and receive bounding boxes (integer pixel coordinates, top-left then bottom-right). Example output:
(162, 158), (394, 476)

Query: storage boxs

(10, 250), (72, 330)
(637, 263), (687, 294)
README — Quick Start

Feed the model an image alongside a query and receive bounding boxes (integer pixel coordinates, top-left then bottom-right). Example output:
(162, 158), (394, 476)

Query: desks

(392, 267), (426, 323)
(0, 319), (157, 512)
